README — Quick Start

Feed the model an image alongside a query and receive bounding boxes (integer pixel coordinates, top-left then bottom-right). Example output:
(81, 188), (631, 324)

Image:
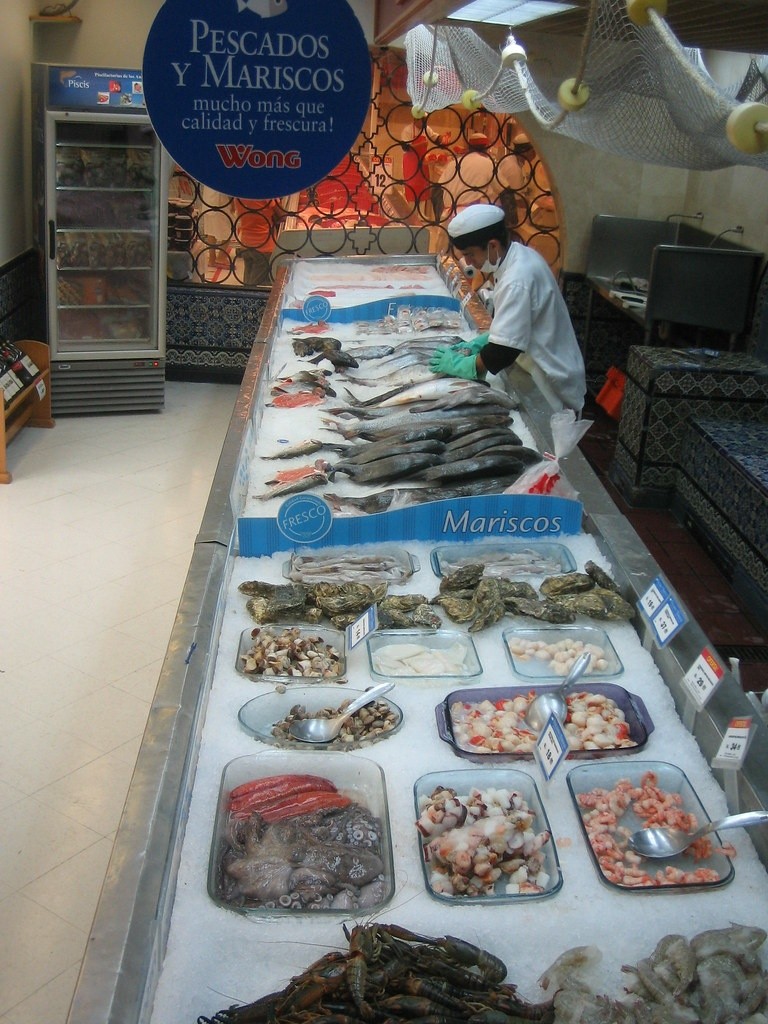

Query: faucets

(707, 224), (745, 249)
(665, 211), (705, 224)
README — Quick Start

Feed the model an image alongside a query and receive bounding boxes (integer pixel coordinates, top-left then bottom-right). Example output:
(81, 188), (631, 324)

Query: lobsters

(197, 874), (563, 1024)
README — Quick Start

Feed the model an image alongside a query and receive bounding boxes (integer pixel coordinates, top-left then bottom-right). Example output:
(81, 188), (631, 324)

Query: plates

(205, 749), (396, 923)
(281, 547), (421, 587)
(565, 760), (736, 896)
(238, 685), (404, 753)
(501, 624), (625, 680)
(435, 682), (655, 759)
(235, 623), (348, 686)
(430, 540), (577, 580)
(413, 767), (564, 908)
(366, 628), (483, 679)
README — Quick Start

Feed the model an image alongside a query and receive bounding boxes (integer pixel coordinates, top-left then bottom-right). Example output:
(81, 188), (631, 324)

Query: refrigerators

(30, 60), (170, 416)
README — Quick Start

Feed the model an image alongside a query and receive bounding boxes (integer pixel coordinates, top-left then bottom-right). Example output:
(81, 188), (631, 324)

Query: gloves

(449, 332), (490, 355)
(428, 346), (487, 380)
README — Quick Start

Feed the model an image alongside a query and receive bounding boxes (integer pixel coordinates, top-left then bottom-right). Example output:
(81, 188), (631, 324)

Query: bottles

(0, 339), (41, 411)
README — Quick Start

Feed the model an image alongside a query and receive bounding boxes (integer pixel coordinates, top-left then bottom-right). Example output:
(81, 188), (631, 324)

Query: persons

(201, 185), (237, 270)
(400, 109), (448, 223)
(430, 203), (587, 421)
(431, 131), (519, 253)
(496, 132), (536, 250)
(233, 194), (286, 286)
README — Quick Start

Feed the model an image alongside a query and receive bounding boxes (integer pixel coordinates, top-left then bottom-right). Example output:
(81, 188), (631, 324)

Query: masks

(479, 246), (501, 272)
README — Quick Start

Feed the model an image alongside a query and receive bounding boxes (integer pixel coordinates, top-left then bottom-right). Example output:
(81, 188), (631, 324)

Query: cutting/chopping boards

(611, 290), (647, 308)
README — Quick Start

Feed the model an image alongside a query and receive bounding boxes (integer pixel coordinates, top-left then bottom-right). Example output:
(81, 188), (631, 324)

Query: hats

(448, 204), (505, 237)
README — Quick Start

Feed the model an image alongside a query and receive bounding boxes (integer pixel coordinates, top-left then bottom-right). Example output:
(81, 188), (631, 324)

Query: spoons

(626, 810), (768, 858)
(287, 681), (396, 744)
(525, 651), (593, 734)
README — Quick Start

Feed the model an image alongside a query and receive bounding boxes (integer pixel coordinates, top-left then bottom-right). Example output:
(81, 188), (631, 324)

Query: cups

(168, 196), (200, 252)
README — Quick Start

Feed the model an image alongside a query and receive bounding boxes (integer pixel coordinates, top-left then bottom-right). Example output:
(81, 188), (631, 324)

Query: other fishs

(252, 308), (543, 517)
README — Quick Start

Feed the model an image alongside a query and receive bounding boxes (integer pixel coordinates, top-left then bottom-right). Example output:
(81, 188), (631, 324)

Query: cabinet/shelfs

(27, 61), (165, 417)
(0, 339), (57, 486)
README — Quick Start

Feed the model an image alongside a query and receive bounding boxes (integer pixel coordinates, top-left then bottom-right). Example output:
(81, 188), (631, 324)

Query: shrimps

(537, 921), (768, 1024)
(574, 769), (737, 887)
